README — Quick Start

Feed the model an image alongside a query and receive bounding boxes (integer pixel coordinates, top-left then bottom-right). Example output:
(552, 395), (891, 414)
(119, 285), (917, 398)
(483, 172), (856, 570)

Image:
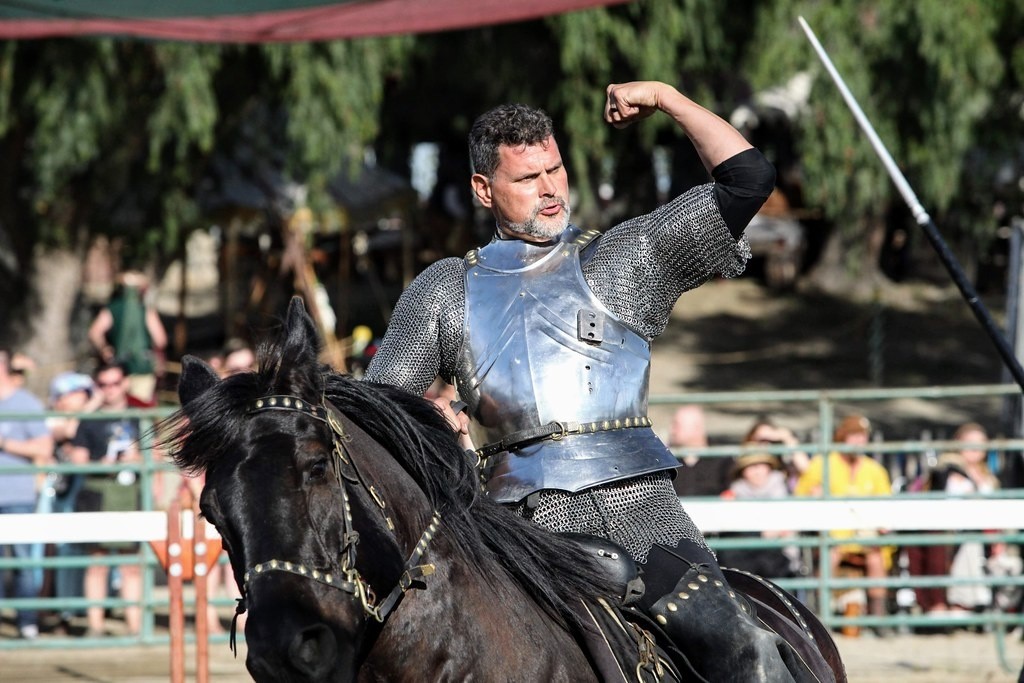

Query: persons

(743, 413), (810, 496)
(721, 440), (790, 551)
(359, 81), (777, 683)
(911, 423), (1007, 623)
(796, 411), (899, 636)
(68, 365), (150, 633)
(656, 403), (738, 497)
(0, 347), (53, 640)
(184, 331), (472, 628)
(87, 271), (166, 458)
(43, 372), (115, 630)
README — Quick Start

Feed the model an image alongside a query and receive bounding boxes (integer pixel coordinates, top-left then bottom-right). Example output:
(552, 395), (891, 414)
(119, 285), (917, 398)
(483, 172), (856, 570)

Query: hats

(728, 441), (781, 470)
(831, 411), (872, 435)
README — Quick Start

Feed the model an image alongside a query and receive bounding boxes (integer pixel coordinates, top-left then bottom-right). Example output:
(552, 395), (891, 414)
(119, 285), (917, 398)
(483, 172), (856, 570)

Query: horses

(128, 294), (850, 683)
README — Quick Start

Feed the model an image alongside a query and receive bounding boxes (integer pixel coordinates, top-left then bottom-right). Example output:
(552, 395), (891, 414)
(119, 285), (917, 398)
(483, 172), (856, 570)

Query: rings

(609, 104), (617, 110)
